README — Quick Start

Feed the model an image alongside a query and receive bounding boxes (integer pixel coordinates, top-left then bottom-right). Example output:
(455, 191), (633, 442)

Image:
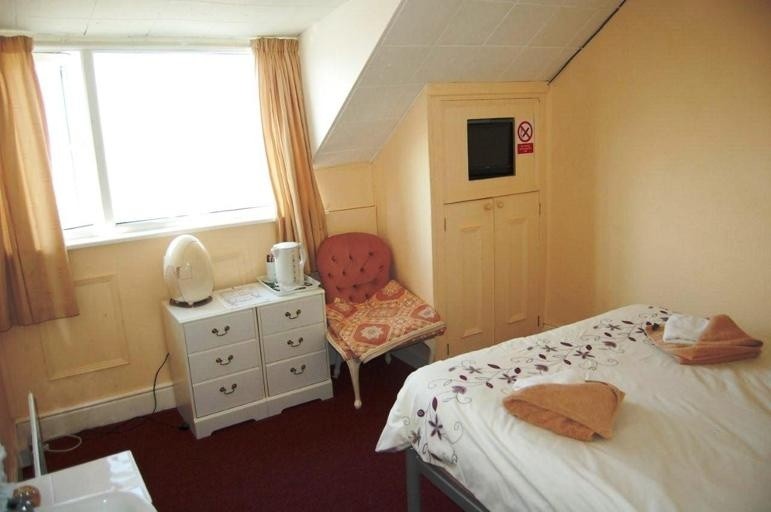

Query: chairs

(318, 232), (447, 412)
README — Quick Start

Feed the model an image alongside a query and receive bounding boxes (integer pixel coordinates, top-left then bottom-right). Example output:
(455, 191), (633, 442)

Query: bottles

(266, 254), (276, 282)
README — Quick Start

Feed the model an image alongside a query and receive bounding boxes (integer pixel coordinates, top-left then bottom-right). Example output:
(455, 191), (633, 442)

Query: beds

(405, 301), (770, 511)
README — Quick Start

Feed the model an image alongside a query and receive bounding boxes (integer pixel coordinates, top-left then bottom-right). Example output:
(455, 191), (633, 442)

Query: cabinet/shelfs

(161, 283), (334, 440)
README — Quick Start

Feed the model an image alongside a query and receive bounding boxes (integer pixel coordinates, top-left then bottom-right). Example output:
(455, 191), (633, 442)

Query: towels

(504, 367), (626, 442)
(645, 313), (762, 365)
